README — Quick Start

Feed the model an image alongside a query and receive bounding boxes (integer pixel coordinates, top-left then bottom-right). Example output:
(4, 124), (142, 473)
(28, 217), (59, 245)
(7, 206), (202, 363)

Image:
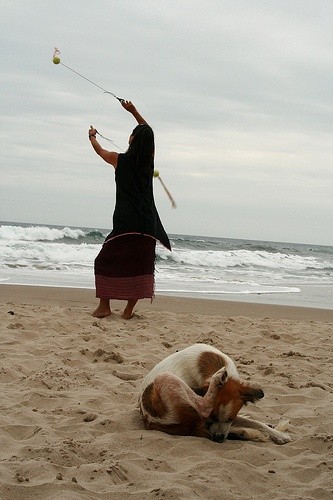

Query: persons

(88, 98), (170, 320)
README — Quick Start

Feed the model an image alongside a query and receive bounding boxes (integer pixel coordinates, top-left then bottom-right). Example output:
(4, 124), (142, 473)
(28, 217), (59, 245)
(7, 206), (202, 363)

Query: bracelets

(88, 134), (96, 141)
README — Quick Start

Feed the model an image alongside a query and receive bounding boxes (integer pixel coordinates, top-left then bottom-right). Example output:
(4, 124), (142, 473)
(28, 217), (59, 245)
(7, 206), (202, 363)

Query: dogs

(138, 344), (294, 445)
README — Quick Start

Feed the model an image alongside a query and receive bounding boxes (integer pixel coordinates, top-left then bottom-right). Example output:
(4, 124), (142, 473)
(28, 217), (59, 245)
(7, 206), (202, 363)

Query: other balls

(154, 171), (159, 176)
(53, 57), (61, 64)
